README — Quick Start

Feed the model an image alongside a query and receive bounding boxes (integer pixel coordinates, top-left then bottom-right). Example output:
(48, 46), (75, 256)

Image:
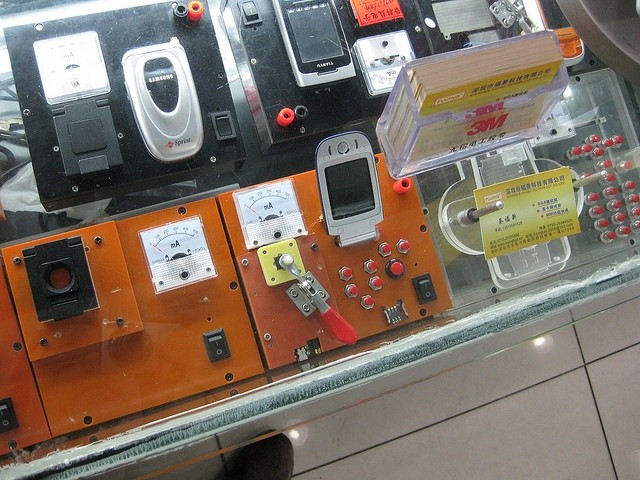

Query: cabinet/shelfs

(0, 0), (640, 480)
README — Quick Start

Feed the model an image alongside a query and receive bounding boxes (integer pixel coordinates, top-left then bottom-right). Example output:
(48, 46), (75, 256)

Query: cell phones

(120, 36), (205, 163)
(313, 130), (385, 249)
(274, 0), (358, 88)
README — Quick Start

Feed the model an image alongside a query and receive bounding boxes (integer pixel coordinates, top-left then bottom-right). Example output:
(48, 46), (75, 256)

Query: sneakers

(214, 429), (294, 480)
